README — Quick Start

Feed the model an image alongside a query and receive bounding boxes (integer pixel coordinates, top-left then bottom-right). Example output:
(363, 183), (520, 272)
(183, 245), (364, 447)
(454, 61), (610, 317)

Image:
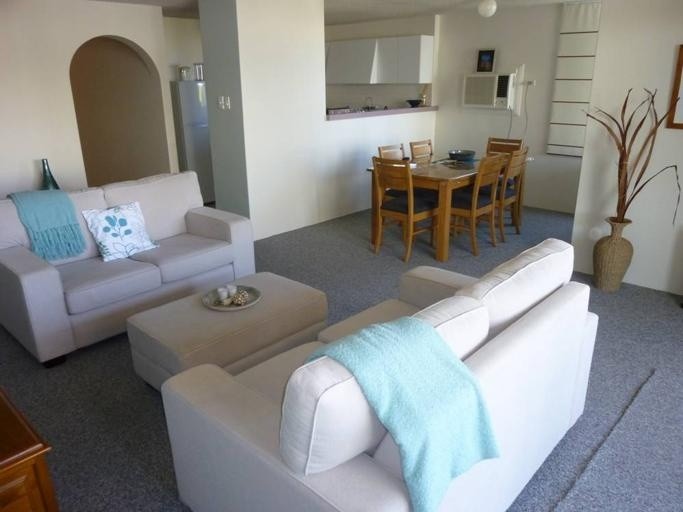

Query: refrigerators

(170, 80), (215, 204)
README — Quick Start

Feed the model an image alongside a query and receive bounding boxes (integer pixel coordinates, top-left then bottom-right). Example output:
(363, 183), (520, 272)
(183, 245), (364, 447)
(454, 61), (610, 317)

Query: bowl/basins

(406, 100), (421, 107)
(447, 150), (475, 160)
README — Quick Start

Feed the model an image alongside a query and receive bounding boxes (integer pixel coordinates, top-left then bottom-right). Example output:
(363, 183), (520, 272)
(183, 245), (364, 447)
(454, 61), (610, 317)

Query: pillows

(79, 200), (156, 265)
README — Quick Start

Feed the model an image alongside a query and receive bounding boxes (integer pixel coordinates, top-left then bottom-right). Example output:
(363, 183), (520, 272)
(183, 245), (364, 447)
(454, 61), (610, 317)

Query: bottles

(422, 95), (427, 107)
(178, 63), (203, 80)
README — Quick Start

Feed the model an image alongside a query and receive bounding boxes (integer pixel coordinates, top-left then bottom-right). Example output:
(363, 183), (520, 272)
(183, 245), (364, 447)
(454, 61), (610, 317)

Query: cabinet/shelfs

(376, 35), (434, 85)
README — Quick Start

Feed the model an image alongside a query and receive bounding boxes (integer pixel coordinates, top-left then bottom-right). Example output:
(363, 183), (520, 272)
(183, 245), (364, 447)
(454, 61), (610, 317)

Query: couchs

(0, 170), (263, 371)
(159, 237), (601, 506)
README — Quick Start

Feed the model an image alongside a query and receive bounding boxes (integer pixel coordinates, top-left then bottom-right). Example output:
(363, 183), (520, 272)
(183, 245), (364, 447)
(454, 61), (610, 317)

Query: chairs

(366, 131), (532, 264)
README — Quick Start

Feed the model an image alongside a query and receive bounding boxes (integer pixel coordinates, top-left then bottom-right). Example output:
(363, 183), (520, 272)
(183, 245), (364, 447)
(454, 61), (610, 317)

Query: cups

(216, 288), (228, 301)
(227, 284), (237, 296)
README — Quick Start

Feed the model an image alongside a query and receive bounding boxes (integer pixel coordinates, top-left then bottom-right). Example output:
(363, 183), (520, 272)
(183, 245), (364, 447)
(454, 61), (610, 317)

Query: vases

(589, 214), (646, 299)
